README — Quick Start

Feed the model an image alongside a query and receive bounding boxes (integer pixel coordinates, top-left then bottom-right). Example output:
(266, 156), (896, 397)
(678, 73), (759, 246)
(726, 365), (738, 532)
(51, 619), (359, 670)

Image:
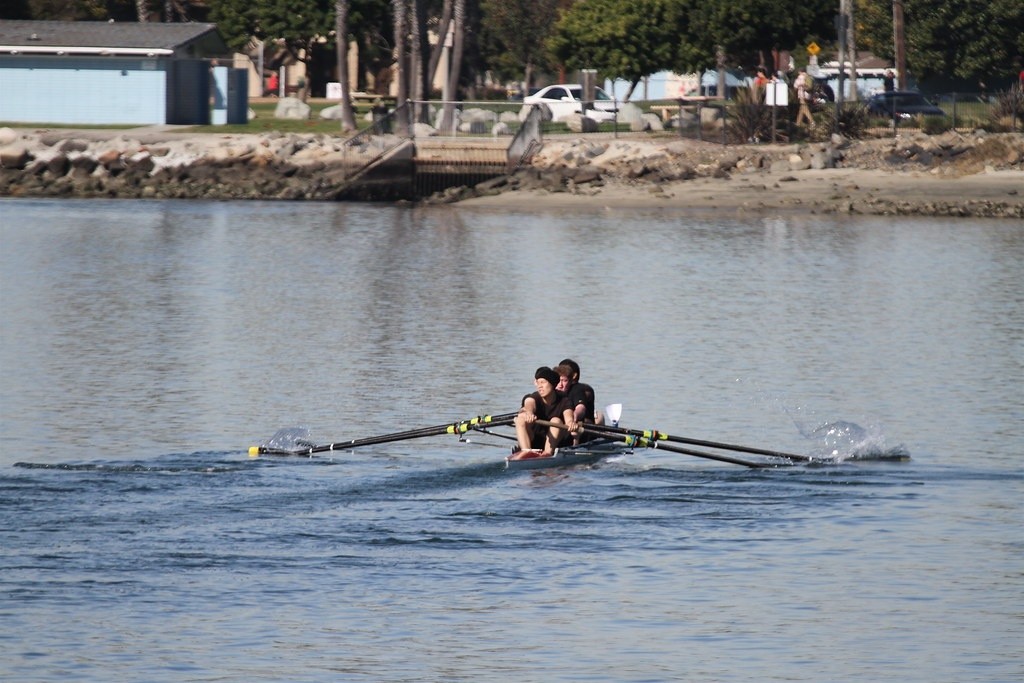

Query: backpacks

(803, 74), (815, 91)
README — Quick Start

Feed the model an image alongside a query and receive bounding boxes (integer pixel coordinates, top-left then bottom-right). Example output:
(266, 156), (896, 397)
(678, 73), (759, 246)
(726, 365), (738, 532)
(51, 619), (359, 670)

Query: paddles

(247, 412), (519, 457)
(530, 415), (911, 467)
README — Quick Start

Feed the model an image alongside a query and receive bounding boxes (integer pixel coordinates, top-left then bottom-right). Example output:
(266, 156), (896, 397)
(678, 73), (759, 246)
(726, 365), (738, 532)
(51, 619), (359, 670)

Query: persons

(268, 71), (279, 97)
(756, 69), (768, 85)
(974, 78), (987, 103)
(593, 409), (605, 425)
(553, 366), (587, 445)
(522, 367), (551, 406)
(516, 370), (579, 454)
(559, 359), (595, 425)
(792, 68), (816, 128)
(210, 58), (222, 103)
(770, 72), (778, 82)
(883, 72), (894, 91)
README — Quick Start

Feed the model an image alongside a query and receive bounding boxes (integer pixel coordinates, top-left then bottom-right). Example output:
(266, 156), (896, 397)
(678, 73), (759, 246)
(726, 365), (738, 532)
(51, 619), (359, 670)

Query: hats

(537, 370), (560, 388)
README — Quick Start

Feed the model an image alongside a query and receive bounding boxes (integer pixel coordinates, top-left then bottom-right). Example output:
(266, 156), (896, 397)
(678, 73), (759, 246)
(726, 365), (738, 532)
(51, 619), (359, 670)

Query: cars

(862, 90), (947, 124)
(262, 69), (279, 96)
(520, 82), (627, 126)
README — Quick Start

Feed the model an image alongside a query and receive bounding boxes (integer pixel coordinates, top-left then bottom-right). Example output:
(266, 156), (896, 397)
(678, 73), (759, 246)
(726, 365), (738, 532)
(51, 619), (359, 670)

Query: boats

(504, 403), (623, 468)
